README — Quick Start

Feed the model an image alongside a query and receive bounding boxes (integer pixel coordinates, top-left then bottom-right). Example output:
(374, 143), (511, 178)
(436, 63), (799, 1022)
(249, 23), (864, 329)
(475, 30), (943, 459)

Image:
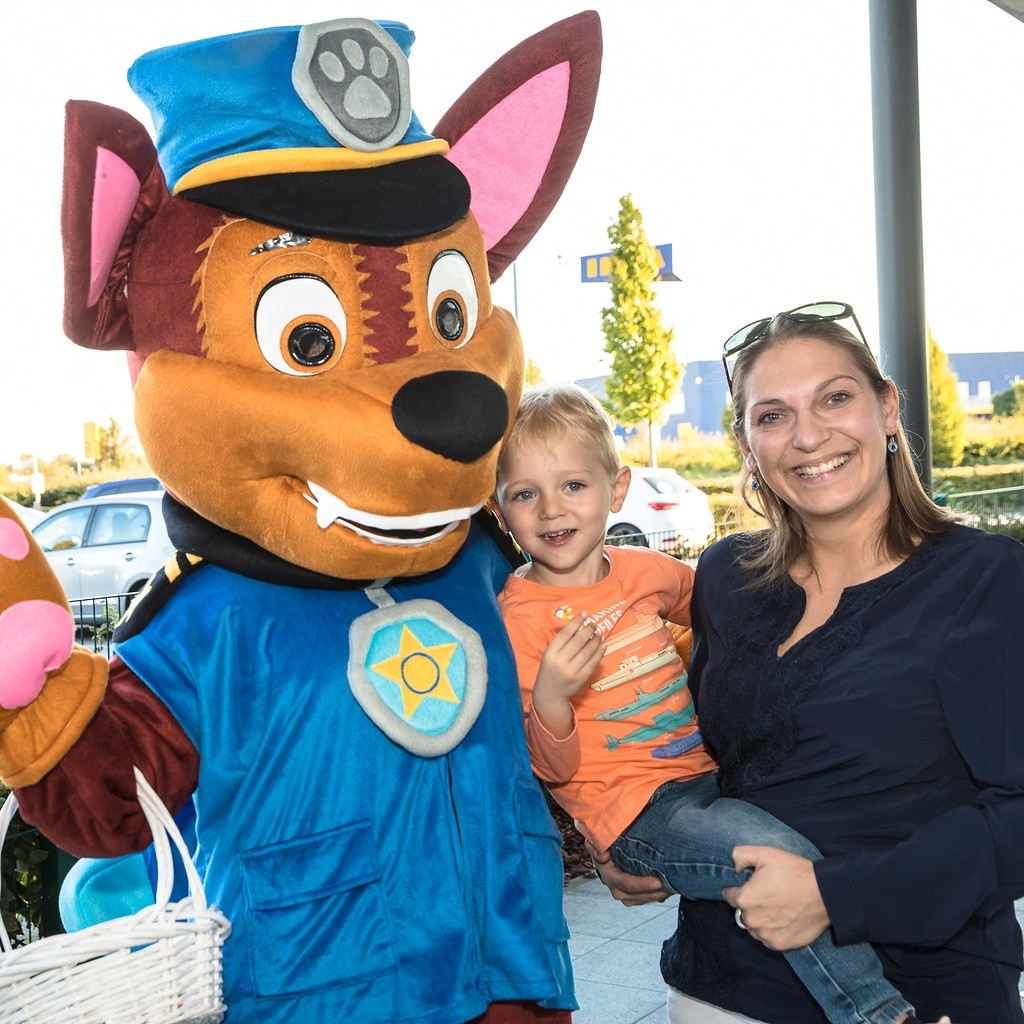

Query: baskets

(0, 765), (233, 1024)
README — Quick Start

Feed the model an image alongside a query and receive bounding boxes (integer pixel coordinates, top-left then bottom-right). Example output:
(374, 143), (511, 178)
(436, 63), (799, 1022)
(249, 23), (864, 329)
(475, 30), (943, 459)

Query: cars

(605, 463), (717, 559)
(29, 490), (179, 638)
(77, 479), (164, 502)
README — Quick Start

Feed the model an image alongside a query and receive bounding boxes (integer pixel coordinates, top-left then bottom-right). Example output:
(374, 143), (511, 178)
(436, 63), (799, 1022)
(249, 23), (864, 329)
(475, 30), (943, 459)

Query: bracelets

(591, 856), (605, 886)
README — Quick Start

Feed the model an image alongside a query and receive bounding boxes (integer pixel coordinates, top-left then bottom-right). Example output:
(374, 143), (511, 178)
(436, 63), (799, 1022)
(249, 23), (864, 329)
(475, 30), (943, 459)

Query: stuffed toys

(2, 11), (600, 1024)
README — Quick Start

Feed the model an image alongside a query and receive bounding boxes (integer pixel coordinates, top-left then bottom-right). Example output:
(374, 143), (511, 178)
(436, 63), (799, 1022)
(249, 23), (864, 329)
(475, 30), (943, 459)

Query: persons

(486, 313), (1024, 1023)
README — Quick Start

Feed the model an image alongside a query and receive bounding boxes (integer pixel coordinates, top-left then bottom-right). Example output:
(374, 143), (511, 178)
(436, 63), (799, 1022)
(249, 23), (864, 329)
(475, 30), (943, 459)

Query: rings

(734, 908), (750, 931)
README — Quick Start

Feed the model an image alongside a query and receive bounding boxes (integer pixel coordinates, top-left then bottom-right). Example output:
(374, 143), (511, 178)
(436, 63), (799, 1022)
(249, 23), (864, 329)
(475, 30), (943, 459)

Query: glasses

(722, 301), (877, 406)
